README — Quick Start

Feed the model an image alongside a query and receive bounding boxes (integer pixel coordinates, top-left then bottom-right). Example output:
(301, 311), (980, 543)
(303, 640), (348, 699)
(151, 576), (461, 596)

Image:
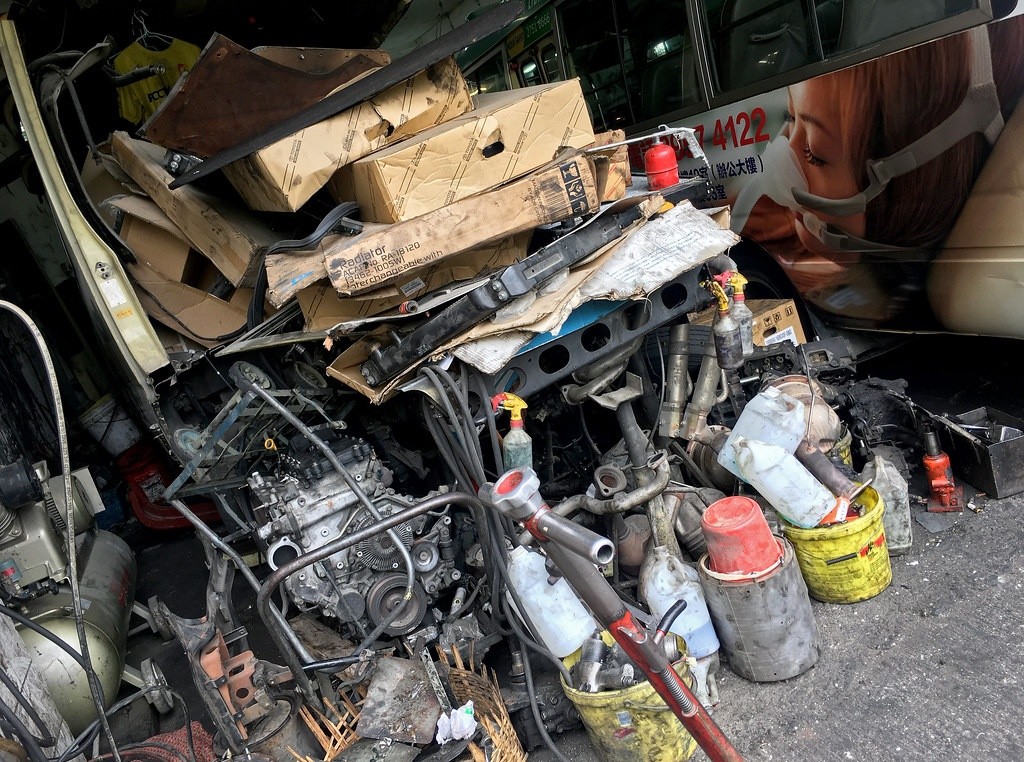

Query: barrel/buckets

(77, 392), (172, 507)
(825, 424), (853, 471)
(700, 496), (785, 583)
(779, 481), (893, 604)
(560, 629), (699, 761)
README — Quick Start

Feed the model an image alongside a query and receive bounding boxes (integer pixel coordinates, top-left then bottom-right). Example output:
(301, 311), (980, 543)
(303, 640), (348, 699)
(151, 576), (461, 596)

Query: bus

(453, 1), (1023, 366)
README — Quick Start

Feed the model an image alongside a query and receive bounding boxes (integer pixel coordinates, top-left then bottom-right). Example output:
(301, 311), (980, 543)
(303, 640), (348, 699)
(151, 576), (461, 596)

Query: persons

(700, 17), (1024, 321)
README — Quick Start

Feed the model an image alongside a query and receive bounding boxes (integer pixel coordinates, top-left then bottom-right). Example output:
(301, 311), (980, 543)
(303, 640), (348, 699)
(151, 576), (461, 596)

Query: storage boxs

(885, 388), (1024, 500)
(692, 297), (808, 349)
(84, 45), (630, 395)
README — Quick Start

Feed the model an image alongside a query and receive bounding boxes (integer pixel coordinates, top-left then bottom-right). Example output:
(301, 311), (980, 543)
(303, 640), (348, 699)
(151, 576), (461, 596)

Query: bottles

(862, 456), (913, 557)
(643, 543), (720, 660)
(717, 386), (837, 529)
(506, 543), (597, 657)
